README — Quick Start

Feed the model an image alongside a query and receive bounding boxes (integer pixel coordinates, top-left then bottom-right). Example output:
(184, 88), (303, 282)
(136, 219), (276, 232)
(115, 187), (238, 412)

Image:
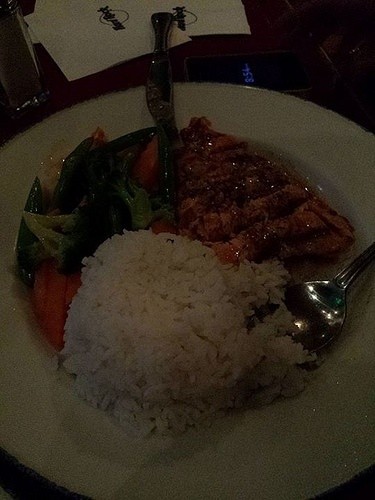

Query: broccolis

(23, 153), (176, 273)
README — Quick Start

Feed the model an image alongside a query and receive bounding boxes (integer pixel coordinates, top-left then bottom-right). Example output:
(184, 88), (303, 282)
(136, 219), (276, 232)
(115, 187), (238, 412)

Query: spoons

(250, 241), (374, 354)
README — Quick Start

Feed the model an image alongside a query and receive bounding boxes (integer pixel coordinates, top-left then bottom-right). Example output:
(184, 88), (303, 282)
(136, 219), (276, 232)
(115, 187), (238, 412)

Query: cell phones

(185, 48), (313, 95)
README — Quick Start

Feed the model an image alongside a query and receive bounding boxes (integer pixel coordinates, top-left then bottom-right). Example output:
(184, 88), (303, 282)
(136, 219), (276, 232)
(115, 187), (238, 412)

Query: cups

(0, 1), (51, 120)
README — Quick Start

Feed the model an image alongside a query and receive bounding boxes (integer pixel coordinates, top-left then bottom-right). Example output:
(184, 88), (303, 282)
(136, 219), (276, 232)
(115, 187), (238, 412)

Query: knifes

(147, 12), (182, 145)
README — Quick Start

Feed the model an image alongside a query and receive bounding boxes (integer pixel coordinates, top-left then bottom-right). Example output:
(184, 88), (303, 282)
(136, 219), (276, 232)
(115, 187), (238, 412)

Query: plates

(0, 83), (374, 500)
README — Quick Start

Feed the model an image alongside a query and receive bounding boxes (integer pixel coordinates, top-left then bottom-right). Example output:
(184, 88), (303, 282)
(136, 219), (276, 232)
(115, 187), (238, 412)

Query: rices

(62, 229), (319, 430)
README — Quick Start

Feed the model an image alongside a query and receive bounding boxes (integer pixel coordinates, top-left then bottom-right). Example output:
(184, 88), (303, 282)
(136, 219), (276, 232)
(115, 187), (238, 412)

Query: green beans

(14, 121), (173, 287)
